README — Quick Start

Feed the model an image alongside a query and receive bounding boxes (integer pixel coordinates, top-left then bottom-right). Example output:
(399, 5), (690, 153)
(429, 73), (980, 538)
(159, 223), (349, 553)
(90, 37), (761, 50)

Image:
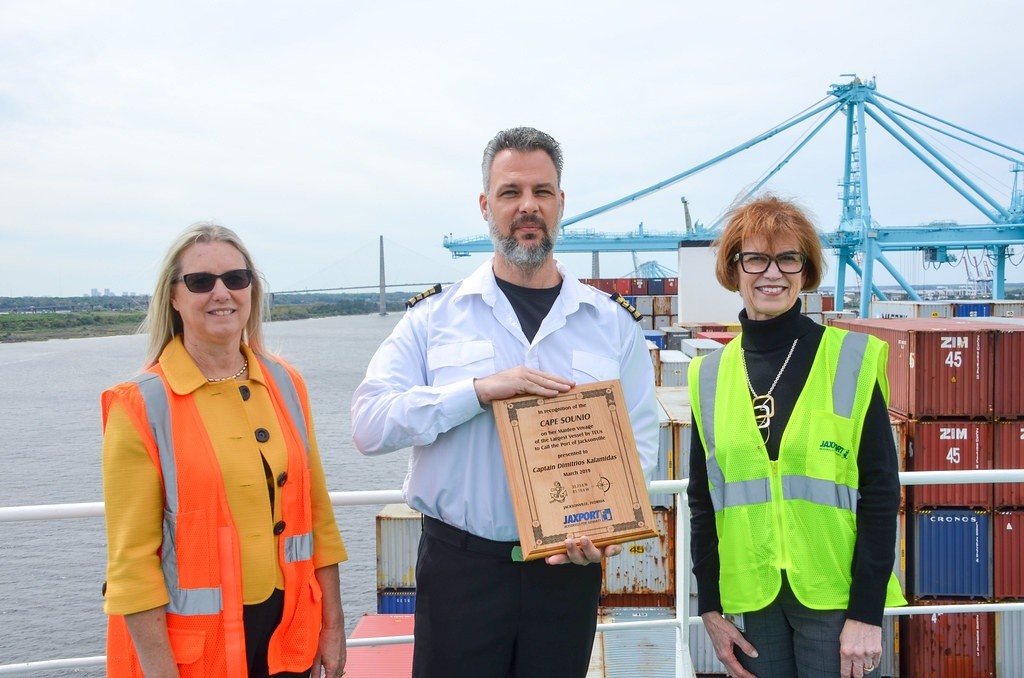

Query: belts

(423, 515), (521, 558)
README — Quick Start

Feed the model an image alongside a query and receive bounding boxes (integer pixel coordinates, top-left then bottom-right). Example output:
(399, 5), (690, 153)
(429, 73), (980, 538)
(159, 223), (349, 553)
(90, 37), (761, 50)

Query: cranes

(444, 72), (1024, 331)
(962, 256), (1007, 296)
(616, 251), (680, 278)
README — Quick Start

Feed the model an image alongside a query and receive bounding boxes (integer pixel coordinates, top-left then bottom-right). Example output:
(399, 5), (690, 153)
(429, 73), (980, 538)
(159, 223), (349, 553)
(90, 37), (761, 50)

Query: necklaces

(202, 357), (249, 385)
(740, 335), (799, 445)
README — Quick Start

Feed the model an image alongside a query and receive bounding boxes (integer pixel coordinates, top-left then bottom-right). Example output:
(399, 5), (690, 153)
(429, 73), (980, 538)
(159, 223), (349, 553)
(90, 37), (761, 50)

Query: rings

(863, 665), (877, 672)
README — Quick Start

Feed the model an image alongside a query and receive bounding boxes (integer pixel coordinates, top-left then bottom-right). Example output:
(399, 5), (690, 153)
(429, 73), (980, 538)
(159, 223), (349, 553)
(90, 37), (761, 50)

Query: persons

(100, 221), (346, 677)
(349, 126), (664, 678)
(688, 197), (900, 678)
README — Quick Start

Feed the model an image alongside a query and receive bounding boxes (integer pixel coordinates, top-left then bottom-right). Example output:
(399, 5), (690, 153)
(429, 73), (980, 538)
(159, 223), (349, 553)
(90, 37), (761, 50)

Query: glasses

(170, 269), (252, 293)
(731, 251), (807, 274)
